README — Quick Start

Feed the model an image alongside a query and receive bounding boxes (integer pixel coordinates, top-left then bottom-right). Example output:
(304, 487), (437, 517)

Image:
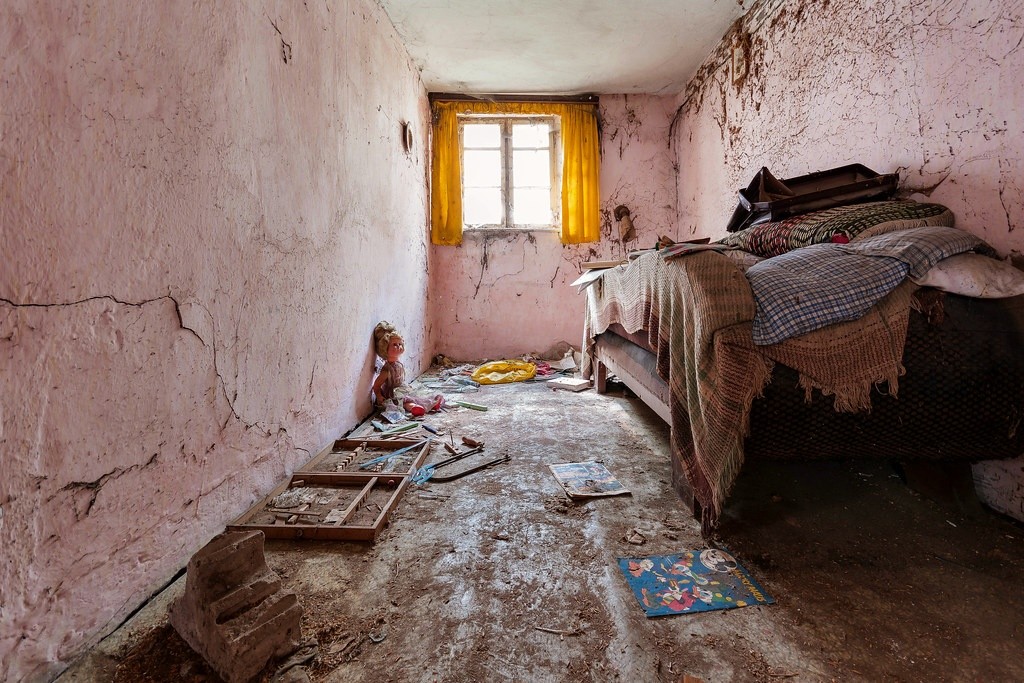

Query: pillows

(913, 252), (1023, 297)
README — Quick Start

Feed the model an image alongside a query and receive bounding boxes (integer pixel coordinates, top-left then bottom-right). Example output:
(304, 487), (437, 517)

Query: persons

(372, 320), (444, 416)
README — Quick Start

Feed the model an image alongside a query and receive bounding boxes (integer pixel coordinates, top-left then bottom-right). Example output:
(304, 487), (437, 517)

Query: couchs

(589, 287), (1024, 483)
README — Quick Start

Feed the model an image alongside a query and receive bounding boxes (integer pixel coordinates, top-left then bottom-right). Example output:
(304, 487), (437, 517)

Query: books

(547, 459), (633, 499)
